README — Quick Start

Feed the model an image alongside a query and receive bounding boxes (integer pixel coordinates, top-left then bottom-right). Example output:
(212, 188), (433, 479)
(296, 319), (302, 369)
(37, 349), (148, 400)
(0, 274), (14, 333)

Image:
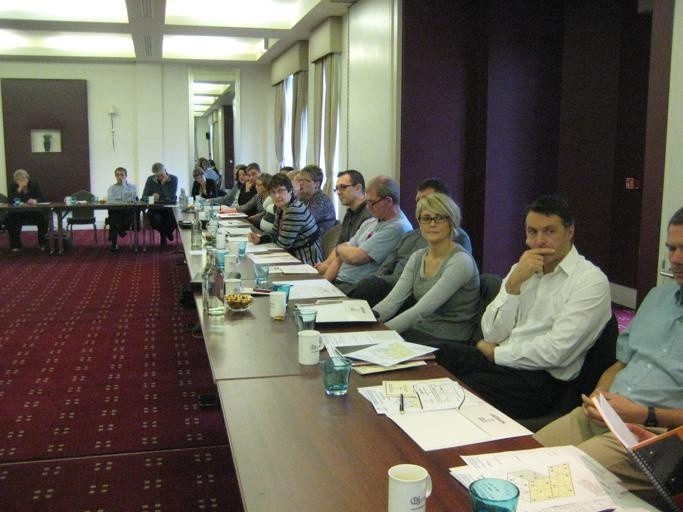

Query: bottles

(178, 187), (225, 319)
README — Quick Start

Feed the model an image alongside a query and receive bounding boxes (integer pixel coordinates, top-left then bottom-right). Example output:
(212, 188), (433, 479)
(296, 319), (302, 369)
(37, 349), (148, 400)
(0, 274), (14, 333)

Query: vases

(43, 135), (51, 151)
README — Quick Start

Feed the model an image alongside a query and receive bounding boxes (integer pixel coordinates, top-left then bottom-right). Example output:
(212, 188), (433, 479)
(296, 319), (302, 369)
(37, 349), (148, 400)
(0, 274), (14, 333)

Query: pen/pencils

(256, 290), (271, 292)
(399, 393), (405, 411)
(220, 202), (222, 211)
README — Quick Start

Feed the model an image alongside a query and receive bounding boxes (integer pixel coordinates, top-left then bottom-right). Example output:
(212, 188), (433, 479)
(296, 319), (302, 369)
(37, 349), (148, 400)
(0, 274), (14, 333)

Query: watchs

(646, 405), (657, 431)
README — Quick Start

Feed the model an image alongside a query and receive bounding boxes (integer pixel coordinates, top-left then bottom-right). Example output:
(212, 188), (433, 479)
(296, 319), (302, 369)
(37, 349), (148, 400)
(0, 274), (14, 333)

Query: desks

(0, 201), (218, 256)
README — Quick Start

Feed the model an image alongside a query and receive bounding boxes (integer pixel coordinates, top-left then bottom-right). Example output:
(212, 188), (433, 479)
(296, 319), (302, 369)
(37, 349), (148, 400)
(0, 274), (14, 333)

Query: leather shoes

(168, 233), (174, 241)
(118, 230), (126, 238)
(111, 243), (119, 251)
(160, 236), (167, 246)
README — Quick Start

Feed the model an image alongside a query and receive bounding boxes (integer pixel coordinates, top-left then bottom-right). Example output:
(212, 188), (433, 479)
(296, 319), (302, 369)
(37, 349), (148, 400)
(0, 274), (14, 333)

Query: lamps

(105, 105), (117, 136)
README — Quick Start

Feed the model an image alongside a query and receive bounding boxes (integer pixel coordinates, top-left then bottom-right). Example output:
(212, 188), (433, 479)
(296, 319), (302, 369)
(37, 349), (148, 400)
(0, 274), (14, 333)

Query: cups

(144, 196), (155, 204)
(64, 196), (76, 205)
(187, 194), (352, 397)
(469, 478), (520, 512)
(387, 464), (433, 512)
(13, 198), (21, 206)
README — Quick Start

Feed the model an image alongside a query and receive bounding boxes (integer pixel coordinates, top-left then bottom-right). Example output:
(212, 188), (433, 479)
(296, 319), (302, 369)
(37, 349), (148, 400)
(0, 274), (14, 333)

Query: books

(592, 393), (683, 508)
(335, 340), (437, 367)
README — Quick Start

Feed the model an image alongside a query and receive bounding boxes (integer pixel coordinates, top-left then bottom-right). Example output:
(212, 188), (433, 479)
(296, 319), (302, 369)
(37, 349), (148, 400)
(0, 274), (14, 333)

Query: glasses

(366, 197), (384, 209)
(418, 216), (447, 224)
(333, 184), (352, 192)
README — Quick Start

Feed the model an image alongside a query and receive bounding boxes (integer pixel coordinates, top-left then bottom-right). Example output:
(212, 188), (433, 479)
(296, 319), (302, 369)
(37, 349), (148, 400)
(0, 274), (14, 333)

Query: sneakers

(10, 248), (20, 252)
(40, 246), (45, 252)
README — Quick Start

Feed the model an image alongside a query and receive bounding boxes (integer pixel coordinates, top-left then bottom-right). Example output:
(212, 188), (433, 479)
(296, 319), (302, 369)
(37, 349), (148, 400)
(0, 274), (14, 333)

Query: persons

(324, 173), (414, 295)
(625, 423), (661, 445)
(191, 154), (337, 271)
(373, 190), (481, 371)
(7, 168), (51, 253)
(348, 179), (474, 318)
(107, 168), (137, 249)
(533, 207), (682, 500)
(314, 170), (374, 275)
(434, 194), (614, 421)
(141, 163), (179, 246)
